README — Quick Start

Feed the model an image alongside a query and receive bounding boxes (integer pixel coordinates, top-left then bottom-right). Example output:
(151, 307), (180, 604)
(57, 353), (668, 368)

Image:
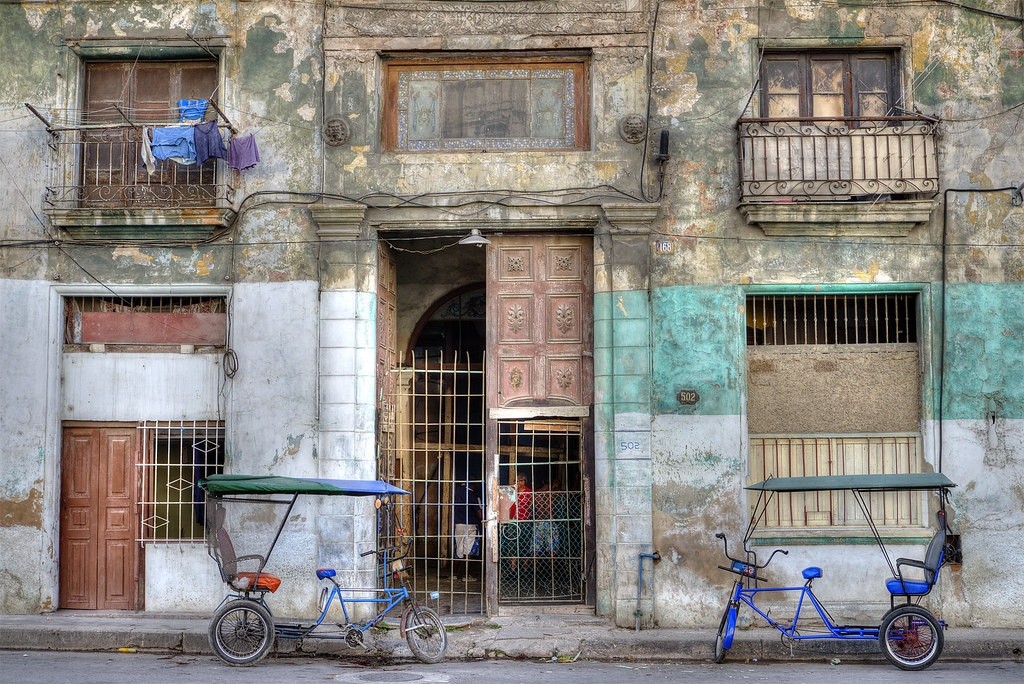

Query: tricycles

(713, 472), (957, 671)
(197, 475), (447, 666)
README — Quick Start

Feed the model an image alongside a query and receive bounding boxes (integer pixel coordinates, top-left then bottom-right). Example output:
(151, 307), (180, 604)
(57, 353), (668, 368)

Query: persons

(529, 479), (564, 592)
(508, 474), (533, 579)
(449, 475), (485, 581)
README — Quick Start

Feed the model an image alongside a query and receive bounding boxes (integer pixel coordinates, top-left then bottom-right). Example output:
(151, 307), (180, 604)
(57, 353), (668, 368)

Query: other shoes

(448, 576), (457, 580)
(462, 575), (477, 581)
